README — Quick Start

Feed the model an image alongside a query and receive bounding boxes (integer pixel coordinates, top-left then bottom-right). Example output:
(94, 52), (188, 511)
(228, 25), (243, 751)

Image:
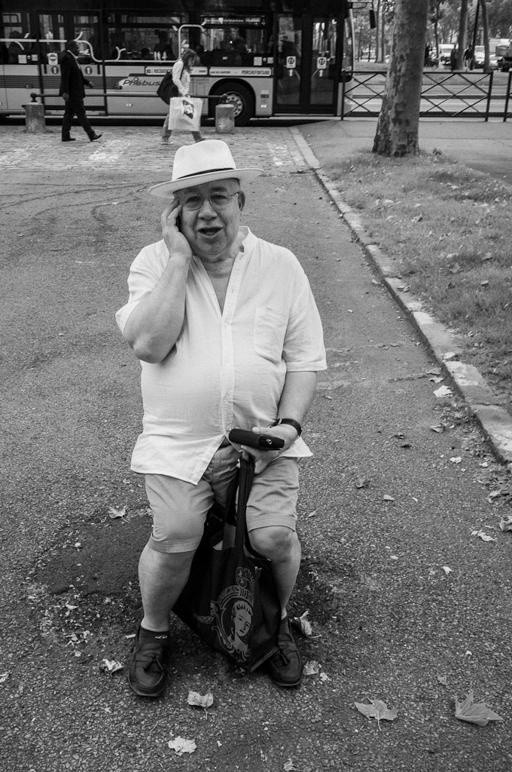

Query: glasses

(173, 191), (239, 211)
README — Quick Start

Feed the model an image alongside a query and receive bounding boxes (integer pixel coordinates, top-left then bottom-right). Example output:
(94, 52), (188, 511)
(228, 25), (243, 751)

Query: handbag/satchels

(168, 97), (204, 133)
(267, 617), (303, 687)
(171, 454), (284, 679)
(157, 72), (180, 104)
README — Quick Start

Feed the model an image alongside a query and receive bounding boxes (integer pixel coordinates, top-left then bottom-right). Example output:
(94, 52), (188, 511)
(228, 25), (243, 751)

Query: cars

(431, 38), (512, 73)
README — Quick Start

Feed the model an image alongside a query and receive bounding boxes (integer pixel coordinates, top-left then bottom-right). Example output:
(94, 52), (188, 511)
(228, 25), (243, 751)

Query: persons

(461, 45), (473, 73)
(56, 41), (105, 143)
(0, 9), (300, 70)
(226, 600), (252, 657)
(113, 135), (330, 699)
(159, 51), (206, 146)
(423, 45), (430, 67)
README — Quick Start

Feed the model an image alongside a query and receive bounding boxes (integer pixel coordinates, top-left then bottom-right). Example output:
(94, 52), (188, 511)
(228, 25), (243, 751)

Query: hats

(147, 139), (264, 199)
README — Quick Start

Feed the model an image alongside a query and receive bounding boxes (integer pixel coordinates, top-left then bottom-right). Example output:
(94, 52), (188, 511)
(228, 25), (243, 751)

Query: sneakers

(126, 619), (175, 698)
(160, 136), (207, 144)
(62, 134), (103, 142)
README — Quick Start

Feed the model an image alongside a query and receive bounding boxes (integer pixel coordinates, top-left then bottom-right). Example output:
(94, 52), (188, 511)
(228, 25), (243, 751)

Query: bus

(1, 1), (377, 127)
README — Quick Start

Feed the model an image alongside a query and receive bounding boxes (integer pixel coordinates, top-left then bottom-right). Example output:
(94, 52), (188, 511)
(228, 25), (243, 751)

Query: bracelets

(272, 417), (303, 437)
